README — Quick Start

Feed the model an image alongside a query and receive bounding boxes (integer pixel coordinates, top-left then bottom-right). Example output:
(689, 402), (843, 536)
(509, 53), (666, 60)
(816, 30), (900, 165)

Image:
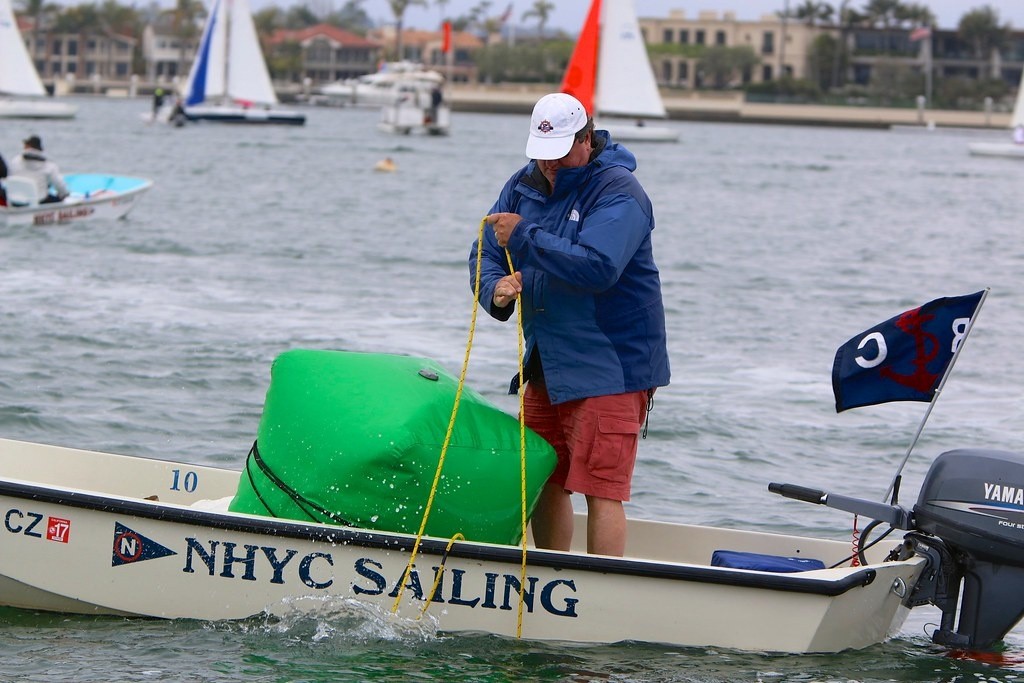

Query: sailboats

(966, 69), (1024, 162)
(563, 1), (681, 144)
(163, 0), (307, 127)
(0, 0), (78, 122)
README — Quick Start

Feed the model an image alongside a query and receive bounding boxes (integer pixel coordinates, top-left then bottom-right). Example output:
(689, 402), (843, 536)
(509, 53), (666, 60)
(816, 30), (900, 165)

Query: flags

(911, 23), (930, 40)
(832, 289), (986, 413)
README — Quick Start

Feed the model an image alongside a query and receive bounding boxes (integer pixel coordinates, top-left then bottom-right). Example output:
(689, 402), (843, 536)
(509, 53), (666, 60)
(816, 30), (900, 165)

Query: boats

(320, 65), (446, 107)
(0, 438), (1024, 658)
(0, 175), (154, 226)
(379, 81), (451, 137)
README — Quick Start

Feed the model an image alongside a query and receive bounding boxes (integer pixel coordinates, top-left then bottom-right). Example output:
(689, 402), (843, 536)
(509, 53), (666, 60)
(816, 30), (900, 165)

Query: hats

(526, 93), (589, 160)
(23, 137), (43, 151)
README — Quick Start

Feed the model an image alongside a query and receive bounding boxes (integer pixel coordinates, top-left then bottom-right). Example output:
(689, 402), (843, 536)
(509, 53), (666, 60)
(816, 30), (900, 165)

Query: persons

(8, 136), (68, 207)
(431, 88), (441, 121)
(169, 100), (184, 121)
(155, 86), (163, 107)
(469, 92), (671, 556)
(915, 92), (927, 122)
(984, 95), (993, 122)
(0, 155), (8, 206)
